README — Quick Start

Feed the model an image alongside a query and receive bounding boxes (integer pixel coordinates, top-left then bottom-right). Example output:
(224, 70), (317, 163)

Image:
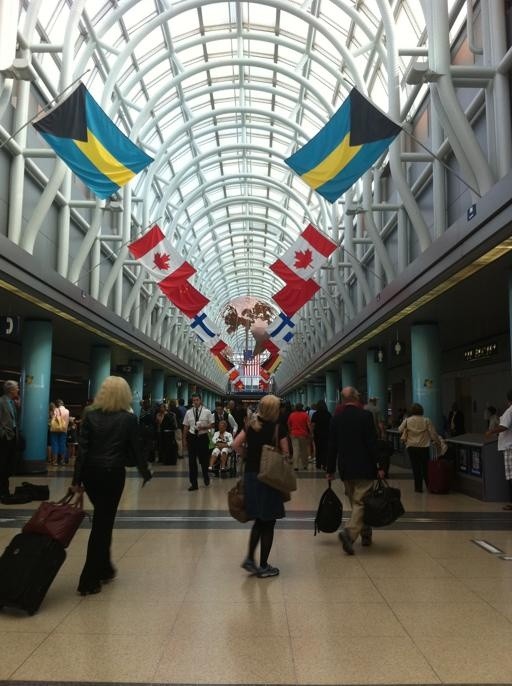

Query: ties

(220, 415), (223, 420)
(194, 408), (199, 435)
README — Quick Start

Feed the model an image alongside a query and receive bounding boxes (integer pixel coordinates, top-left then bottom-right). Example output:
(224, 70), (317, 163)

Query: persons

(228, 394), (293, 579)
(363, 395), (386, 441)
(0, 380), (21, 504)
(447, 401), (464, 435)
(397, 402), (442, 493)
(209, 398), (331, 472)
(484, 389), (512, 509)
(139, 397), (191, 465)
(70, 373), (154, 597)
(49, 397), (70, 466)
(181, 391), (217, 491)
(324, 386), (387, 556)
(487, 405), (499, 430)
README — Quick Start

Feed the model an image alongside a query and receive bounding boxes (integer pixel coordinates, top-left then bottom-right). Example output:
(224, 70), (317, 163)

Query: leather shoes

(188, 486), (198, 491)
(204, 476), (210, 485)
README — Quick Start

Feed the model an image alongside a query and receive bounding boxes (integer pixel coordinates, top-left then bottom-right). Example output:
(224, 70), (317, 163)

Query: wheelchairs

(210, 444), (239, 481)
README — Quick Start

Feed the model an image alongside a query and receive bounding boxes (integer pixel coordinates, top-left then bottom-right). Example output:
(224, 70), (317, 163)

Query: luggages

(425, 439), (454, 497)
(0, 489), (85, 618)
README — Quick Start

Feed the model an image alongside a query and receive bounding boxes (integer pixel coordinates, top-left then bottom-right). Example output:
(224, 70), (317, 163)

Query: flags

(32, 81), (152, 202)
(268, 222), (341, 292)
(282, 86), (403, 203)
(126, 222), (196, 296)
(166, 277), (321, 390)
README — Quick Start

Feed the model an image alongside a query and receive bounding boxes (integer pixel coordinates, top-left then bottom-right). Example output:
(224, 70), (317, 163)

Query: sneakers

(257, 564), (280, 578)
(240, 557), (259, 574)
(339, 528), (355, 555)
(361, 534), (372, 546)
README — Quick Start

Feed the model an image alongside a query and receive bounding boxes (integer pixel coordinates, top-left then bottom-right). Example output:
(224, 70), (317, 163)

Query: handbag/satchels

(314, 479), (342, 536)
(227, 479), (256, 524)
(257, 443), (297, 494)
(49, 416), (68, 433)
(360, 479), (406, 527)
(400, 427), (408, 442)
(20, 500), (86, 549)
(13, 482), (50, 502)
